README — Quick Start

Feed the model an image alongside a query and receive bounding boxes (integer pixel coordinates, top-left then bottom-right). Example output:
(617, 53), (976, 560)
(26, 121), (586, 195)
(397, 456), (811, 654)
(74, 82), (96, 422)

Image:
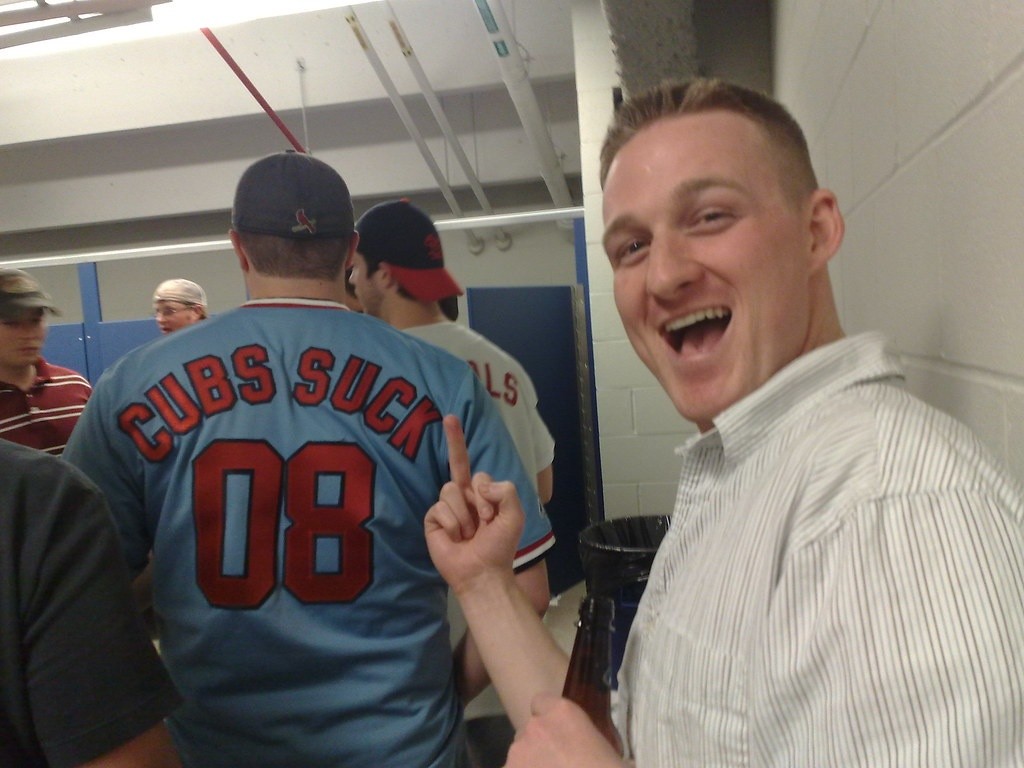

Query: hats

(233, 149), (354, 239)
(153, 278), (208, 319)
(0, 268), (61, 317)
(355, 197), (463, 303)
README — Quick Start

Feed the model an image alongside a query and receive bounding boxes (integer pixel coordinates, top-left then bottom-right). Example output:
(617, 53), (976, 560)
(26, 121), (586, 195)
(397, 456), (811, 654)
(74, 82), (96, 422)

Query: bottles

(562, 591), (626, 761)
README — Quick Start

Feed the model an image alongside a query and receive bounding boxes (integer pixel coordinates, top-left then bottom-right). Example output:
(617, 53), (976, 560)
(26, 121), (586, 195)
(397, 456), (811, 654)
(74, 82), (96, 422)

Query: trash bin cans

(580, 514), (672, 691)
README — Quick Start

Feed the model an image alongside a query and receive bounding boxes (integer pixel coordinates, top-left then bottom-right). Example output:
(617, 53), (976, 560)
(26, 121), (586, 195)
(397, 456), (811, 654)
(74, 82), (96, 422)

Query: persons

(0, 436), (183, 768)
(0, 265), (91, 458)
(424, 77), (1024, 768)
(62, 149), (558, 768)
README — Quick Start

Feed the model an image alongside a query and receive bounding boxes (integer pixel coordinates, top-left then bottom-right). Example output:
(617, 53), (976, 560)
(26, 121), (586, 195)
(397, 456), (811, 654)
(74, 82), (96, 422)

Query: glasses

(155, 302), (204, 317)
